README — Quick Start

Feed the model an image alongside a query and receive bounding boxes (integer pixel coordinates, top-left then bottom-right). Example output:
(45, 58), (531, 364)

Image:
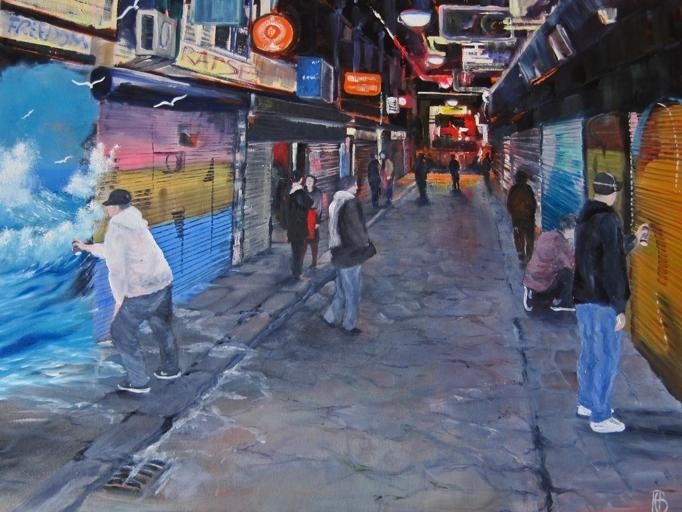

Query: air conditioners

(132, 5), (179, 65)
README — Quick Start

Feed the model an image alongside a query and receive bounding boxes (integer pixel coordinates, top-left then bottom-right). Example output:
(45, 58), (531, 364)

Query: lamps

(395, 1), (460, 108)
